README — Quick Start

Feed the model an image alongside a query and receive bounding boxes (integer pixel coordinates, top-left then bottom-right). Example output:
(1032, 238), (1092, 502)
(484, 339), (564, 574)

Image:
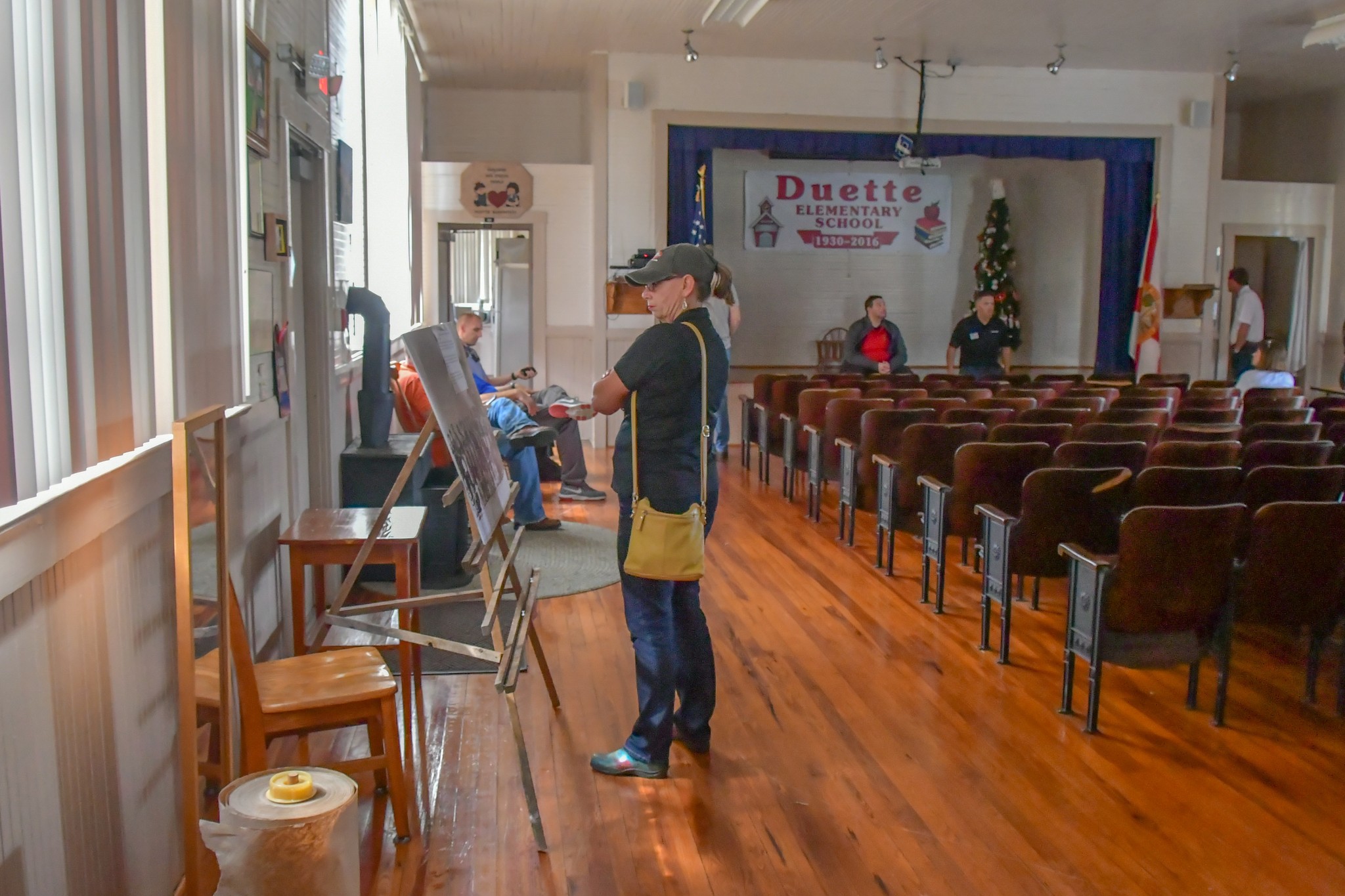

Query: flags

(688, 184), (706, 249)
(1127, 200), (1164, 386)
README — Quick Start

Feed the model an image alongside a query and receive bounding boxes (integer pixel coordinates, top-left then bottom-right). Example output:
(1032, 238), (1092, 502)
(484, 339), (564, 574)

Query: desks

(278, 504), (430, 706)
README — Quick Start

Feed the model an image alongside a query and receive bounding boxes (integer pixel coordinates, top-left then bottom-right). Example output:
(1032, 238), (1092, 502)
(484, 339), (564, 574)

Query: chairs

(820, 326), (850, 362)
(737, 366), (1344, 738)
(190, 638), (225, 804)
(225, 575), (411, 843)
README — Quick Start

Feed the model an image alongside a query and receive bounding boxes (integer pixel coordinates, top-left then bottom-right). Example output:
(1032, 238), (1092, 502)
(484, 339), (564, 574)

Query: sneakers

(548, 397), (598, 420)
(590, 747), (668, 779)
(672, 722), (710, 750)
(559, 483), (607, 501)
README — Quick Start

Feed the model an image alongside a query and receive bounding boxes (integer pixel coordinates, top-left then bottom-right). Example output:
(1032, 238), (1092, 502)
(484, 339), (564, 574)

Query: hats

(626, 243), (715, 287)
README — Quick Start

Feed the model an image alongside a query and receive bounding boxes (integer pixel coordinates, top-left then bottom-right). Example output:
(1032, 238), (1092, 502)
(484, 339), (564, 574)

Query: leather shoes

(515, 518), (561, 531)
(508, 426), (558, 449)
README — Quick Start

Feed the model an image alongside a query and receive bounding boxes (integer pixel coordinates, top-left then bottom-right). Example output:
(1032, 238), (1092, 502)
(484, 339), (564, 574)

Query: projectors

(898, 155), (942, 175)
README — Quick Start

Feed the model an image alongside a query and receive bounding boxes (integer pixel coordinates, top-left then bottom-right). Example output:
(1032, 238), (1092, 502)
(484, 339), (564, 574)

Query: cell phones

(521, 366), (536, 376)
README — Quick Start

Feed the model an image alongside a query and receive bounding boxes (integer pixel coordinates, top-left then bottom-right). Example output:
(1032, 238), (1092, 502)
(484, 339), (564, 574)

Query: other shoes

(711, 446), (727, 461)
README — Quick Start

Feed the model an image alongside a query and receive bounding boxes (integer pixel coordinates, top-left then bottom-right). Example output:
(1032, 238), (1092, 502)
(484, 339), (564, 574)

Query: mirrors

(168, 405), (234, 894)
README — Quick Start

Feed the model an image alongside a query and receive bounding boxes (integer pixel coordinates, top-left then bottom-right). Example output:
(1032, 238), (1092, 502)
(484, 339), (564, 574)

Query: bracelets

(510, 382), (516, 389)
(511, 372), (518, 380)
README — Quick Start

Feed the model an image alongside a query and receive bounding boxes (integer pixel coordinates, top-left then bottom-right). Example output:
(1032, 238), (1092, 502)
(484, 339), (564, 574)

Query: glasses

(645, 275), (680, 291)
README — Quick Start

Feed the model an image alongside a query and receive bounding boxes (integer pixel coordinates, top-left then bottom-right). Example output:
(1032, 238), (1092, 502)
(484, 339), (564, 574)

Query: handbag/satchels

(623, 498), (705, 581)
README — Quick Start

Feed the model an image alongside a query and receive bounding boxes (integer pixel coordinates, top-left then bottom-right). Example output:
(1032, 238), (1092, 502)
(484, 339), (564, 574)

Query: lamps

(1046, 43), (1070, 75)
(1225, 45), (1241, 87)
(871, 35), (889, 68)
(680, 29), (700, 63)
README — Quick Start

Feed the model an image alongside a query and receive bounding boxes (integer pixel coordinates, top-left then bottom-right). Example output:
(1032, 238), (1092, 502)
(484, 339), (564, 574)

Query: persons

(454, 310), (563, 483)
(446, 402), (505, 516)
(1233, 335), (1295, 401)
(945, 290), (1013, 377)
(471, 372), (609, 501)
(388, 321), (562, 532)
(1227, 266), (1265, 384)
(588, 242), (737, 780)
(695, 272), (742, 461)
(838, 294), (913, 375)
(245, 62), (268, 140)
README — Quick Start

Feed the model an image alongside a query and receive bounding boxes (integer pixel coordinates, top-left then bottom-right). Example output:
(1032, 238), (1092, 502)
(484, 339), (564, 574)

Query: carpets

(354, 520), (622, 601)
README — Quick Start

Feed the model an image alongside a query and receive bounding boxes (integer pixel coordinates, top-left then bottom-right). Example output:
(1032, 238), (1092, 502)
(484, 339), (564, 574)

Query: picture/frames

(263, 211), (290, 260)
(245, 24), (274, 159)
